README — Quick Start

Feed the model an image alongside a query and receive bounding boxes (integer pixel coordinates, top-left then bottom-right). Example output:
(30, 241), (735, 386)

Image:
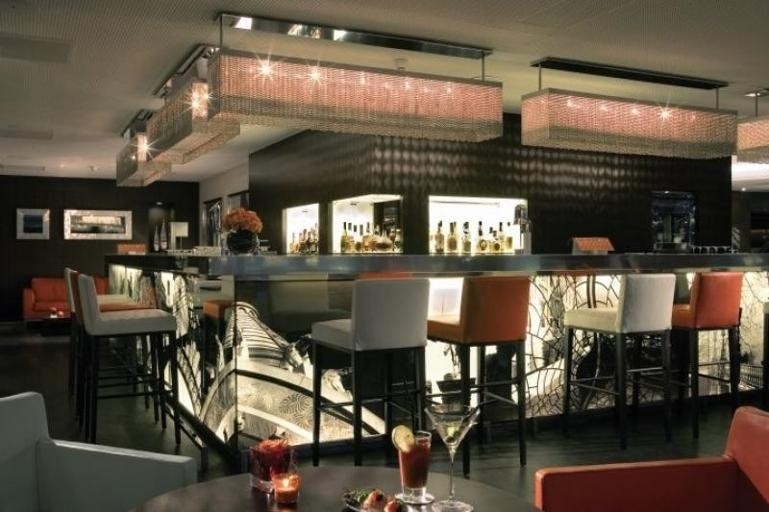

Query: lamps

(116, 108), (173, 189)
(520, 56), (737, 162)
(146, 42), (239, 163)
(208, 15), (504, 144)
(737, 89), (769, 164)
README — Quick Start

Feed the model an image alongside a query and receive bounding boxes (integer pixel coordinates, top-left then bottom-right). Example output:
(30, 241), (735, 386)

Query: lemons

(391, 424), (415, 454)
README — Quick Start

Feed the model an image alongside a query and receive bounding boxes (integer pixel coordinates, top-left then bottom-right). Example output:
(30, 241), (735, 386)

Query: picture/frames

(63, 209), (133, 240)
(15, 208), (49, 240)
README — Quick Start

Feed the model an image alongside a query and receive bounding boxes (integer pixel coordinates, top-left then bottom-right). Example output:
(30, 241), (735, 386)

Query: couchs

(22, 277), (110, 329)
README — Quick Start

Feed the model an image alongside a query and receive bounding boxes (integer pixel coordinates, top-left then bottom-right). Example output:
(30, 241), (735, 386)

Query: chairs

(562, 272), (677, 450)
(429, 273), (531, 477)
(64, 268), (133, 396)
(534, 406), (769, 511)
(1, 392), (196, 512)
(311, 278), (430, 466)
(70, 269), (153, 429)
(77, 272), (182, 446)
(663, 271), (746, 440)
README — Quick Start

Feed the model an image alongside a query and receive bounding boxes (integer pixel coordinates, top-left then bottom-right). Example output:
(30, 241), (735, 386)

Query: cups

(395, 432), (437, 505)
(249, 444), (295, 493)
(271, 473), (300, 505)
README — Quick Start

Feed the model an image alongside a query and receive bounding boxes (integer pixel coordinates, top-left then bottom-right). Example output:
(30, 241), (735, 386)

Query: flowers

(221, 208), (264, 232)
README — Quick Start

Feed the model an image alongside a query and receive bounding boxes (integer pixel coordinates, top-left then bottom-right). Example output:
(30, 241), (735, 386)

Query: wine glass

(424, 404), (477, 512)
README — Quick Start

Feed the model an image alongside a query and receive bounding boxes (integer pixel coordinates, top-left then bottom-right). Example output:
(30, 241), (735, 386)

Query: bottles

(476, 220), (516, 252)
(154, 224), (160, 251)
(290, 226), (320, 255)
(448, 222), (458, 253)
(337, 220), (402, 253)
(435, 221), (445, 253)
(160, 217), (168, 250)
(460, 221), (472, 253)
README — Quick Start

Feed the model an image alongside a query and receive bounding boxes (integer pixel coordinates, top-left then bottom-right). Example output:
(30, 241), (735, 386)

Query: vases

(227, 231), (259, 255)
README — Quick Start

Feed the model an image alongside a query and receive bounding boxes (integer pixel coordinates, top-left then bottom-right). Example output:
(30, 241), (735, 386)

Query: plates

(341, 489), (421, 512)
